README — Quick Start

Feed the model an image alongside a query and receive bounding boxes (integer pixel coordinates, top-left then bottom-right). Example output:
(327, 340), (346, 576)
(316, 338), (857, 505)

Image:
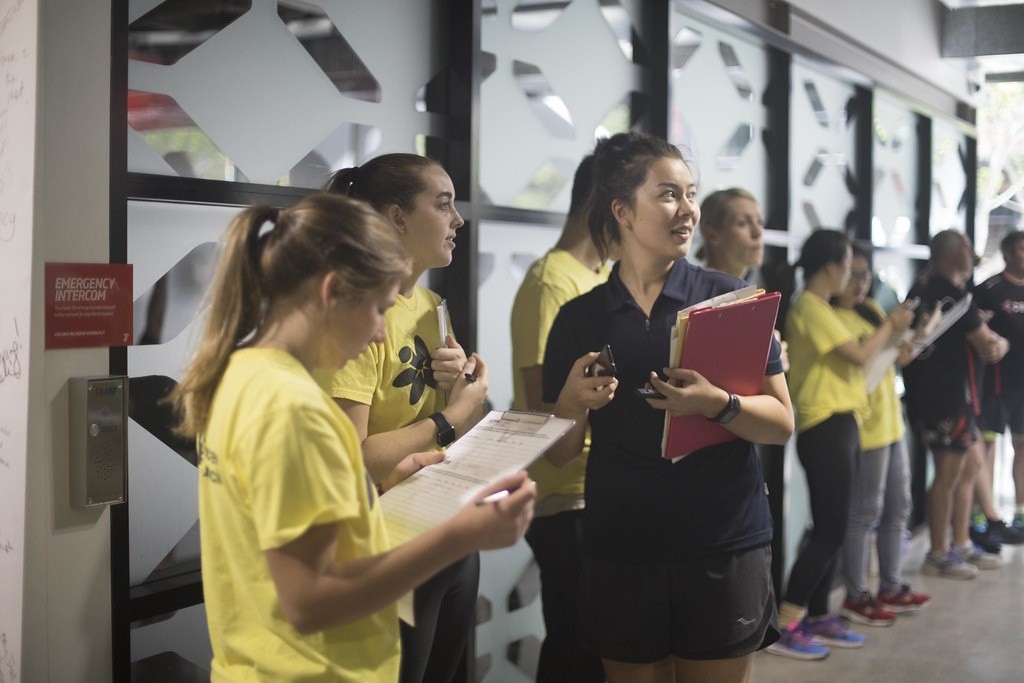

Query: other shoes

(986, 521), (1024, 544)
(971, 513), (988, 533)
(969, 530), (1001, 554)
(1012, 514), (1024, 528)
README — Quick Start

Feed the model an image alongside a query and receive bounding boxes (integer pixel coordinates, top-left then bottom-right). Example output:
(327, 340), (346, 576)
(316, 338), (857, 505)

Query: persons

(508, 144), (628, 683)
(165, 194), (536, 681)
(764, 226), (923, 662)
(530, 134), (798, 682)
(947, 257), (1024, 557)
(974, 230), (1024, 545)
(321, 152), (494, 681)
(692, 186), (790, 374)
(829, 243), (931, 629)
(904, 227), (1002, 583)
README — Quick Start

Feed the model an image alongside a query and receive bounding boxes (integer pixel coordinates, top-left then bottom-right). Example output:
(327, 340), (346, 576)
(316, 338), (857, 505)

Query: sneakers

(802, 614), (867, 648)
(877, 583), (933, 613)
(923, 548), (979, 580)
(951, 544), (1004, 568)
(765, 622), (831, 659)
(841, 590), (896, 627)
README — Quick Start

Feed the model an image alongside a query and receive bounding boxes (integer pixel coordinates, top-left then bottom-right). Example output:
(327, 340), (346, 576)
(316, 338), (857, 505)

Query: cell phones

(589, 345), (617, 390)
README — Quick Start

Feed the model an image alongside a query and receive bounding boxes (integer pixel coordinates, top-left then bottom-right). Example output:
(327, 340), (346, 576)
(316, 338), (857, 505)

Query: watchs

(705, 390), (741, 424)
(427, 411), (456, 448)
